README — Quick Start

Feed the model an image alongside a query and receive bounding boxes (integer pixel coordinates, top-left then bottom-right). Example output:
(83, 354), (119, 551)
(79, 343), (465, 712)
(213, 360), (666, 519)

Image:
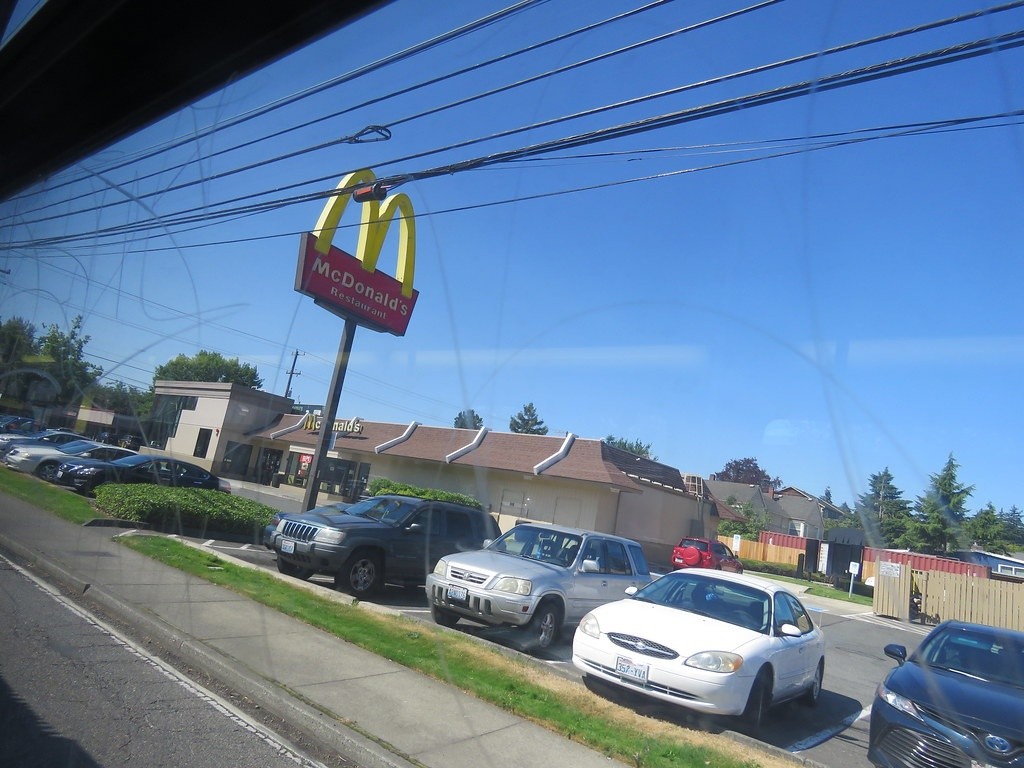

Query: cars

(2, 441), (138, 483)
(0, 413), (95, 458)
(96, 431), (118, 446)
(867, 618), (1024, 768)
(56, 453), (232, 499)
(572, 567), (826, 732)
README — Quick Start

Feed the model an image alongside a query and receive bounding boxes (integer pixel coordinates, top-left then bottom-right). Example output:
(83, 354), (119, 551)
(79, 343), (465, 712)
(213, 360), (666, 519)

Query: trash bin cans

(515, 520), (532, 542)
(272, 474), (281, 489)
(261, 470), (273, 486)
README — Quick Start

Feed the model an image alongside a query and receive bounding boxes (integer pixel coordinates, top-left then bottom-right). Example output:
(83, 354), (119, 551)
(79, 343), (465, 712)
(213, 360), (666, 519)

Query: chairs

(749, 601), (764, 624)
(692, 590), (708, 607)
(530, 539), (555, 561)
(568, 545), (577, 564)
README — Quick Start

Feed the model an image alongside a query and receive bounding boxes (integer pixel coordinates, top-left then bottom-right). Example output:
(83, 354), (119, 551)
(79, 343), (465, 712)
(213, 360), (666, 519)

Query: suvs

(262, 492), (508, 600)
(671, 535), (744, 575)
(118, 434), (141, 451)
(424, 522), (652, 654)
